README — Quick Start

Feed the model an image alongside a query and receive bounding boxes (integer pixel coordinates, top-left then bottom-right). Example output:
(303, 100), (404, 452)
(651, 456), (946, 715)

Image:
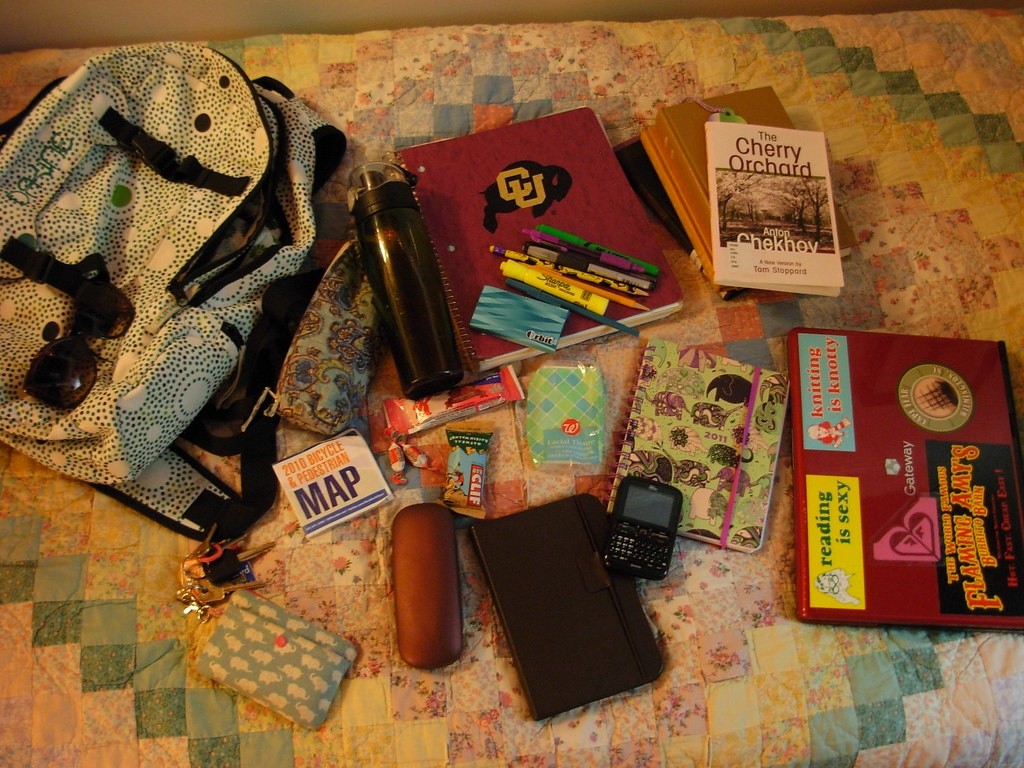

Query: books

(470, 491), (665, 721)
(393, 104), (684, 374)
(605, 335), (791, 555)
(787, 326), (1024, 632)
(638, 84), (860, 303)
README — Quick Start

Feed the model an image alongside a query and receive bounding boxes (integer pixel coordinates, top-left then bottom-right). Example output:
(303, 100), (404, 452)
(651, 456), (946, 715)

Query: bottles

(346, 160), (465, 399)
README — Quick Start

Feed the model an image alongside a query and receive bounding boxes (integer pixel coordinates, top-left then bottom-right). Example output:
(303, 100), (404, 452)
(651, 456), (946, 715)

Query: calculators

(602, 475), (683, 580)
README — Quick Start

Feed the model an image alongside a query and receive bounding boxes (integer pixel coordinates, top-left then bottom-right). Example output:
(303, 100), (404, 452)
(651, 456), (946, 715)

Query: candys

(383, 428), (407, 485)
(381, 364), (524, 437)
(395, 433), (439, 471)
(433, 421), (494, 519)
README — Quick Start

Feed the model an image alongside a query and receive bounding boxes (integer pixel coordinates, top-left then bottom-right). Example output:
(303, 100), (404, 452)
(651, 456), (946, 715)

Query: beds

(2, 1), (1022, 767)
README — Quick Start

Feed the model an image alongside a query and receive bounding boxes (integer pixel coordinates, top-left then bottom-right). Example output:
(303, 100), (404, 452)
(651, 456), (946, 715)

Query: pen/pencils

(488, 223), (661, 337)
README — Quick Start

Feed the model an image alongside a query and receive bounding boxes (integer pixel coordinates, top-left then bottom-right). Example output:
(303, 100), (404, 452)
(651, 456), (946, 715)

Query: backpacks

(0, 44), (347, 542)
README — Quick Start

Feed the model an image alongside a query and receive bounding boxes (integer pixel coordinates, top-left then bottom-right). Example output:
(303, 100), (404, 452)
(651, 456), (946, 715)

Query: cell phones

(603, 473), (682, 580)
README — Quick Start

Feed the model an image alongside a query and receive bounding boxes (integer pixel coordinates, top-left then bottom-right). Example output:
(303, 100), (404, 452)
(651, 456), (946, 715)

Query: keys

(175, 522), (276, 621)
